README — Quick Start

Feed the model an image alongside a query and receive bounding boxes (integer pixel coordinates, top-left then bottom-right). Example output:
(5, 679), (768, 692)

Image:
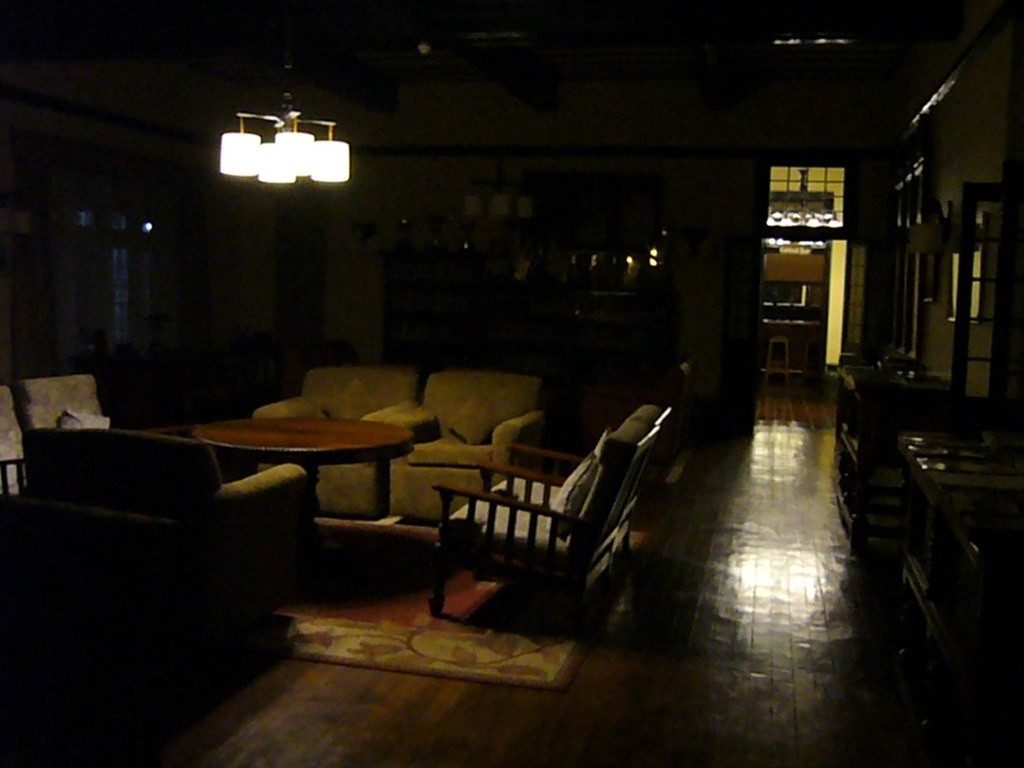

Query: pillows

(546, 428), (610, 534)
(448, 393), (507, 444)
(320, 378), (381, 421)
(60, 410), (110, 432)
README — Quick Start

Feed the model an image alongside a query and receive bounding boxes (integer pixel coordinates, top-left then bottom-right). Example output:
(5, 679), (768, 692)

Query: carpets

(279, 555), (595, 688)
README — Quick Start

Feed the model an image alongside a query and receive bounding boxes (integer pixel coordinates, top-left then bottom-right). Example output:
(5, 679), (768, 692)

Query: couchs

(389, 366), (546, 524)
(580, 361), (693, 467)
(252, 363), (425, 521)
(0, 373), (103, 504)
(283, 339), (357, 402)
(0, 428), (310, 694)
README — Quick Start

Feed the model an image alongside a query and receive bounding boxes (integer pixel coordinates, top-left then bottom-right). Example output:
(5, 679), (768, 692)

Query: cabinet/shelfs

(899, 431), (1024, 735)
(839, 362), (954, 554)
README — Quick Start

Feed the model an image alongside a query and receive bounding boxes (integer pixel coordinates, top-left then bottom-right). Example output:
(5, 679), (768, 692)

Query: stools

(768, 335), (791, 373)
(803, 336), (824, 373)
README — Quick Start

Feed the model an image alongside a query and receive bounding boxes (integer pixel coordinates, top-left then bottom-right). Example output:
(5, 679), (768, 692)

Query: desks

(192, 415), (416, 596)
(763, 317), (822, 370)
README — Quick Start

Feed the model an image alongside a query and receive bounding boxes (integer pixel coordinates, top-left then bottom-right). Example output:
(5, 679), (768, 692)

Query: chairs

(428, 405), (674, 629)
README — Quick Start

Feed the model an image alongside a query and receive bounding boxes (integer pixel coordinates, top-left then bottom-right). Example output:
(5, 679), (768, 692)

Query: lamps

(472, 153), (535, 221)
(217, 0), (350, 187)
